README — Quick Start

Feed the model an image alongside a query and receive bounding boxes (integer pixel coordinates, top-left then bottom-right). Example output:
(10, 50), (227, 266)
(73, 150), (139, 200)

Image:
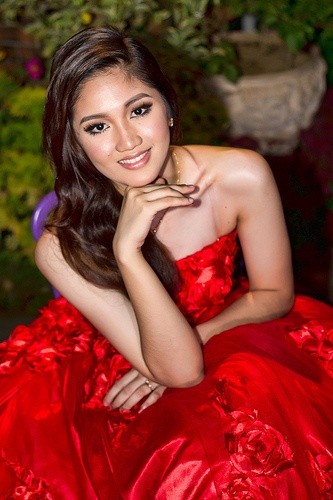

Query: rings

(145, 379), (154, 391)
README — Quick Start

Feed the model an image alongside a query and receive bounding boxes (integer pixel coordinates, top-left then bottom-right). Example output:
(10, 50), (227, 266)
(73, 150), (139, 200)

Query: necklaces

(149, 146), (180, 236)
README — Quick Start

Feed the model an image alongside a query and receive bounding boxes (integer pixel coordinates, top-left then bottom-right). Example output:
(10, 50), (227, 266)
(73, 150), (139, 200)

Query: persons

(0, 24), (333, 500)
